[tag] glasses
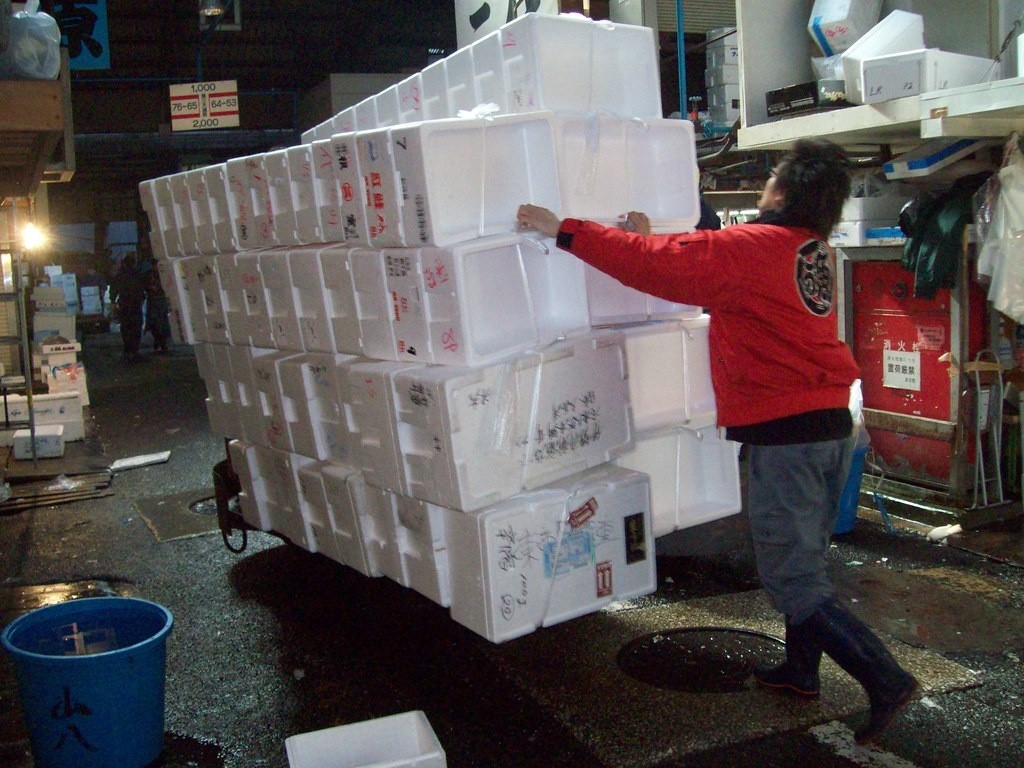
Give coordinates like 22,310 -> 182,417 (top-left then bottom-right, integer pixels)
769,168 -> 778,176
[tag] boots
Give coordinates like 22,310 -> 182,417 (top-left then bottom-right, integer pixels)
800,597 -> 920,746
754,615 -> 823,698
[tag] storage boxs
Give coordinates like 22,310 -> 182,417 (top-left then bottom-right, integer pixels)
0,266 -> 103,459
766,0 -> 994,117
136,13 -> 742,644
827,195 -> 909,249
285,710 -> 447,768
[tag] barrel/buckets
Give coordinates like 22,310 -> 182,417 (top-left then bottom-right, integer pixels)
0,596 -> 174,768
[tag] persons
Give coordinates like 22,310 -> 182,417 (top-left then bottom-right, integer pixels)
112,253 -> 174,364
517,138 -> 919,745
80,266 -> 108,331
